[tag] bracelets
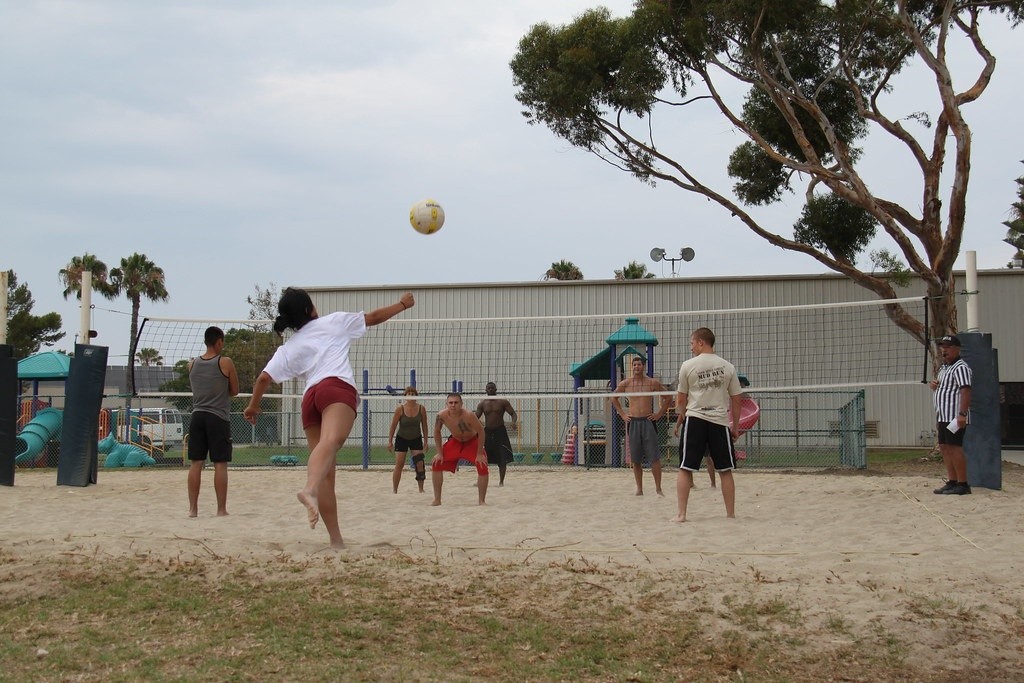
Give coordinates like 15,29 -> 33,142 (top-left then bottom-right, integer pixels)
400,301 -> 407,309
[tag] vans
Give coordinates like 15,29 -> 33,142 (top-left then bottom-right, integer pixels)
108,407 -> 183,451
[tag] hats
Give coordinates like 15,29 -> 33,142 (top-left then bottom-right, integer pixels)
935,335 -> 961,348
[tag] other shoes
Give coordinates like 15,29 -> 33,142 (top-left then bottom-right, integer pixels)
934,479 -> 958,495
942,482 -> 971,495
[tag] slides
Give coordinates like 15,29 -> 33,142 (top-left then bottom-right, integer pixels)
12,408 -> 62,469
731,395 -> 760,459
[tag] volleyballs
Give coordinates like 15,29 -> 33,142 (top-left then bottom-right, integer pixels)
409,199 -> 445,235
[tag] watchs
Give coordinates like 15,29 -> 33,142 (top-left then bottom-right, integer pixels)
959,412 -> 967,416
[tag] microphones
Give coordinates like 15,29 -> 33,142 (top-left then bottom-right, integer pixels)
943,352 -> 947,357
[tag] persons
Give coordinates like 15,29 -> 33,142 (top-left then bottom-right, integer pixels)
674,414 -> 716,488
611,357 -> 672,496
428,393 -> 489,507
388,386 -> 428,494
930,335 -> 971,495
474,382 -> 517,487
671,327 -> 742,522
245,289 -> 415,547
266,425 -> 274,446
187,327 -> 239,516
